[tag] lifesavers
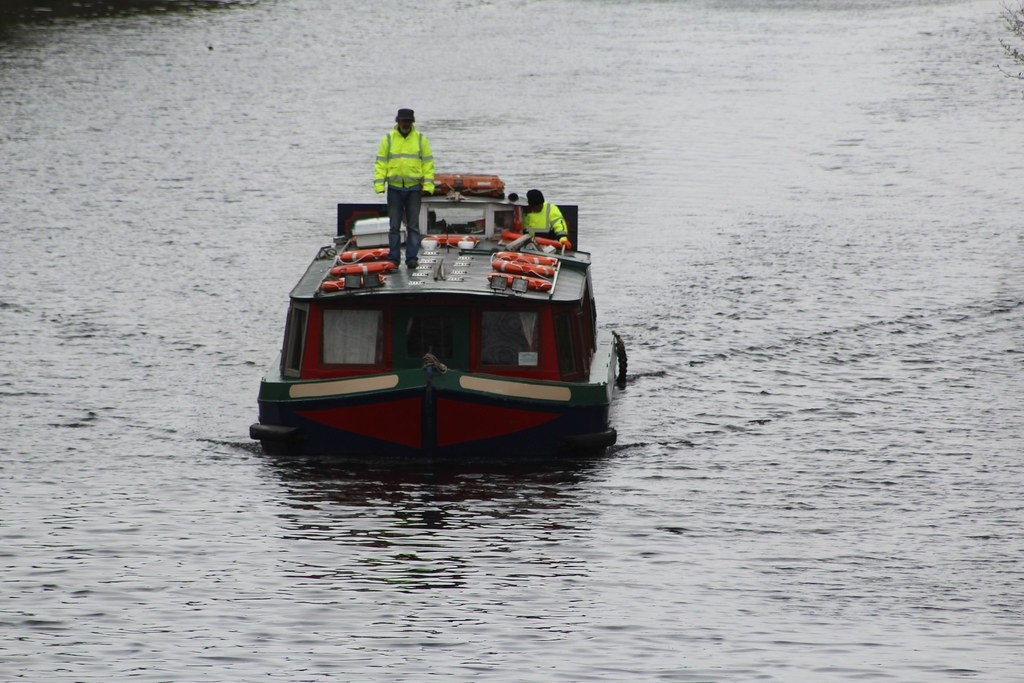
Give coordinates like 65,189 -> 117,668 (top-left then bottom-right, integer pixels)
496,250 -> 559,267
328,261 -> 397,276
493,258 -> 557,280
339,247 -> 391,261
487,272 -> 553,293
501,231 -> 563,251
425,234 -> 480,248
320,275 -> 388,291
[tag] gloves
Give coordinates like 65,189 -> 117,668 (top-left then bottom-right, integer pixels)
559,237 -> 571,249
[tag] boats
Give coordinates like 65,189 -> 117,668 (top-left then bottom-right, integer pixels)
249,174 -> 628,469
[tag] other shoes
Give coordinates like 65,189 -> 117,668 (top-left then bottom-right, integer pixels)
407,259 -> 419,269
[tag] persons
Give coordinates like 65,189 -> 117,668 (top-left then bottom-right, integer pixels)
523,189 -> 572,249
373,109 -> 435,269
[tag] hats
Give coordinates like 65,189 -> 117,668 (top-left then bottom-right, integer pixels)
526,189 -> 544,206
395,108 -> 415,122
